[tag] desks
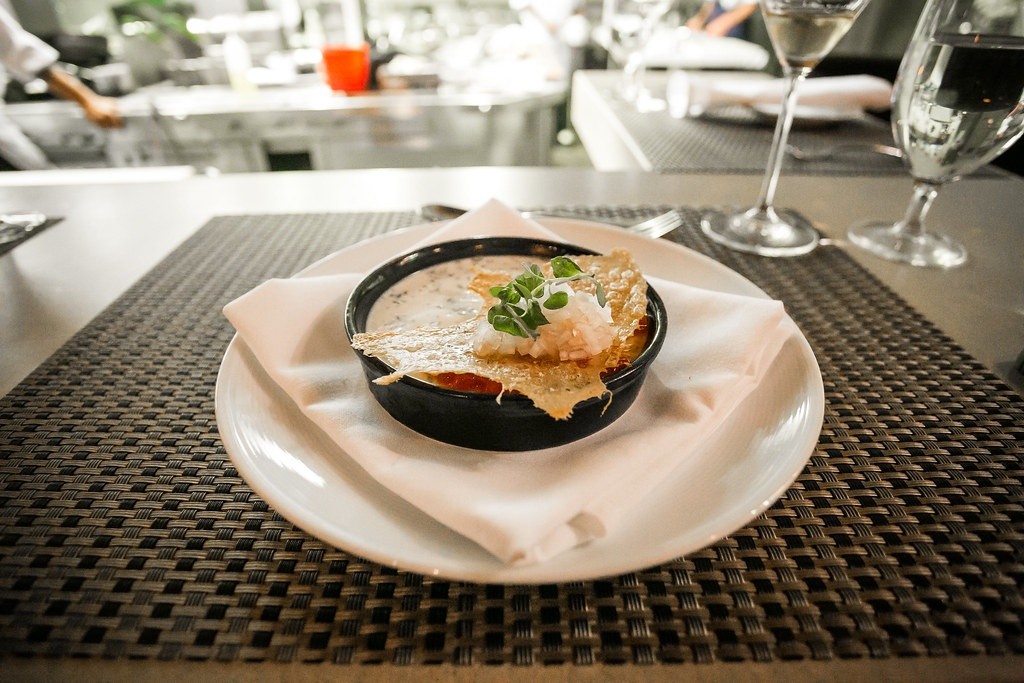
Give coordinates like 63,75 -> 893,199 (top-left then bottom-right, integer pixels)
0,72 -> 1024,683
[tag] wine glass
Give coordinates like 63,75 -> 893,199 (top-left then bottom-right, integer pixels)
847,0 -> 1024,269
702,0 -> 870,258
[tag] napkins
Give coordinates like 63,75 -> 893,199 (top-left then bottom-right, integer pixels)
667,73 -> 894,118
221,195 -> 796,568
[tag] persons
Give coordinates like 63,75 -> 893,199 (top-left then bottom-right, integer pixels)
685,0 -> 760,42
0,4 -> 128,128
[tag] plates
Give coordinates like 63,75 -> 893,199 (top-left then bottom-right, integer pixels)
215,215 -> 825,585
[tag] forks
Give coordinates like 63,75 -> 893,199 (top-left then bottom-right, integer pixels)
421,204 -> 683,238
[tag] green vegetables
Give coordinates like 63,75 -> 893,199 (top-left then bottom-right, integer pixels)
487,256 -> 607,340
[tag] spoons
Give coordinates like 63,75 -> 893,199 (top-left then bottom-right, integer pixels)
763,136 -> 904,162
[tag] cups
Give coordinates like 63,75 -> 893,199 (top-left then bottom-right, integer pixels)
320,43 -> 370,97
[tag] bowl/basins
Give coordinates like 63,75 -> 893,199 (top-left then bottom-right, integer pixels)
342,237 -> 668,453
